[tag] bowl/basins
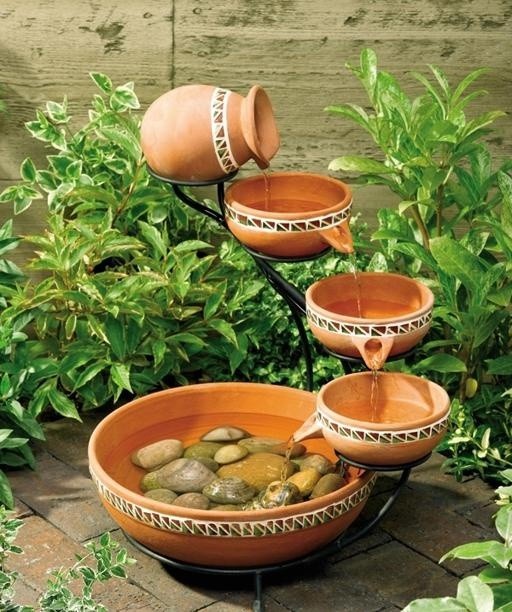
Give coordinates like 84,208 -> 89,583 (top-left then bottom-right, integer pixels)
85,381 -> 381,570
